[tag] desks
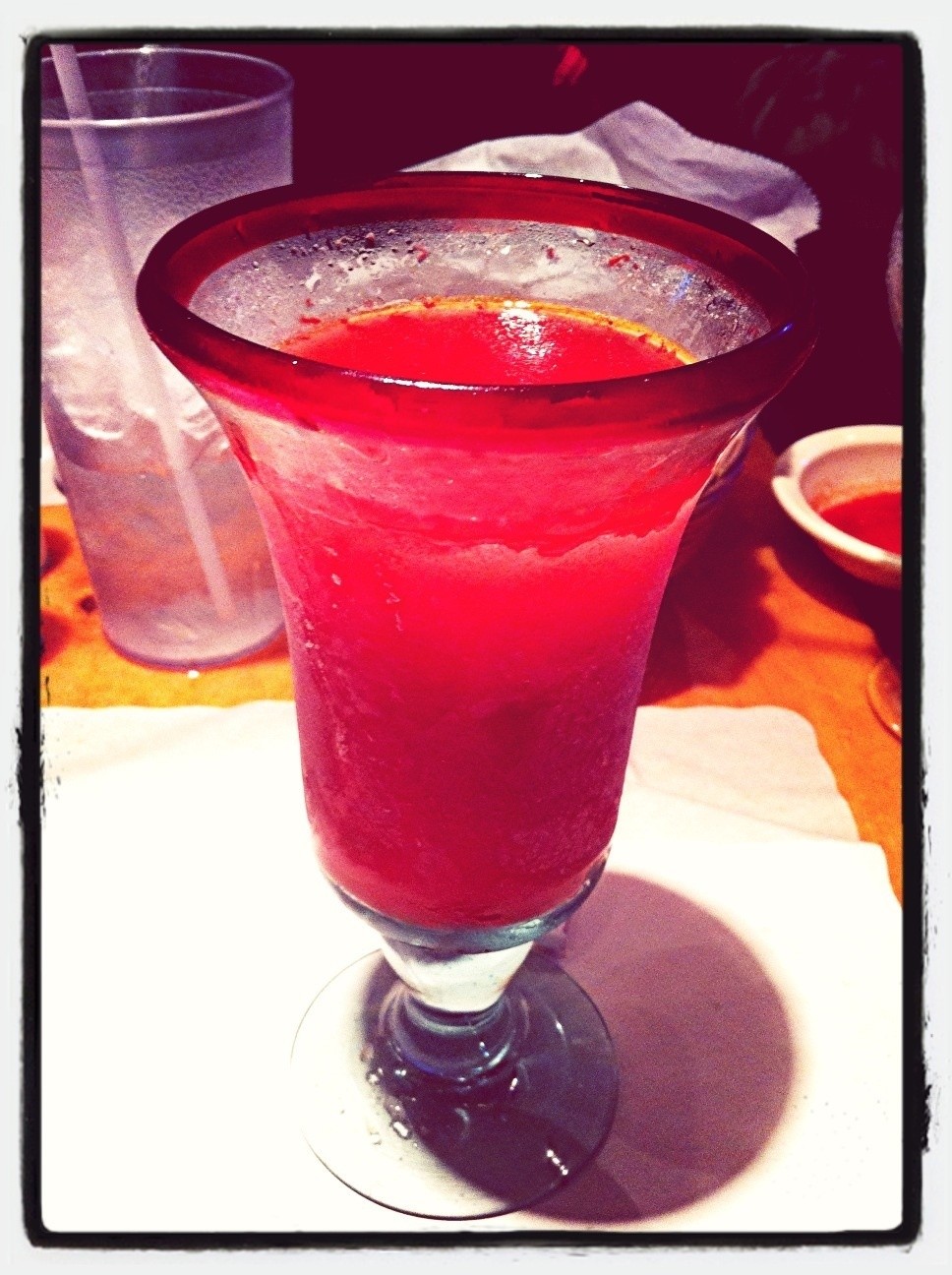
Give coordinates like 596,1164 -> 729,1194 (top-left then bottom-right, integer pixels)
40,417 -> 907,1233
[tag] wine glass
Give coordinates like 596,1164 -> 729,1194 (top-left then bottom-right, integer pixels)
135,169 -> 826,1221
859,210 -> 900,746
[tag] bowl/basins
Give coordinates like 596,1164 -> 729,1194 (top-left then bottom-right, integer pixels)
670,422 -> 750,579
770,425 -> 901,590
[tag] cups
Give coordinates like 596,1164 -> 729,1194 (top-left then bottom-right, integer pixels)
39,50 -> 294,667
